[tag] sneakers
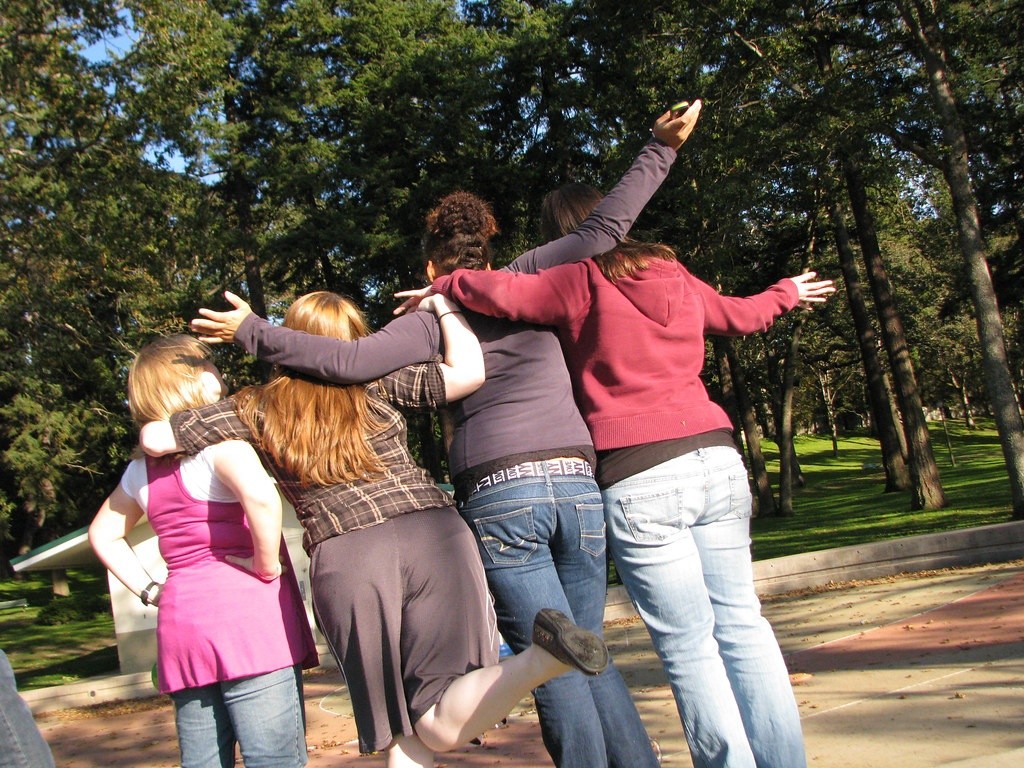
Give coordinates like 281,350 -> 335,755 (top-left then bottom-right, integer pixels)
530,608 -> 609,675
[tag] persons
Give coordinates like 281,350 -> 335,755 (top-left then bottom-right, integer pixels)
393,183 -> 835,768
139,291 -> 608,768
190,98 -> 702,766
88,335 -> 317,767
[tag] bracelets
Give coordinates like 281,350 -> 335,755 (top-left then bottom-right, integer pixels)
436,311 -> 463,322
252,561 -> 281,581
141,581 -> 158,606
